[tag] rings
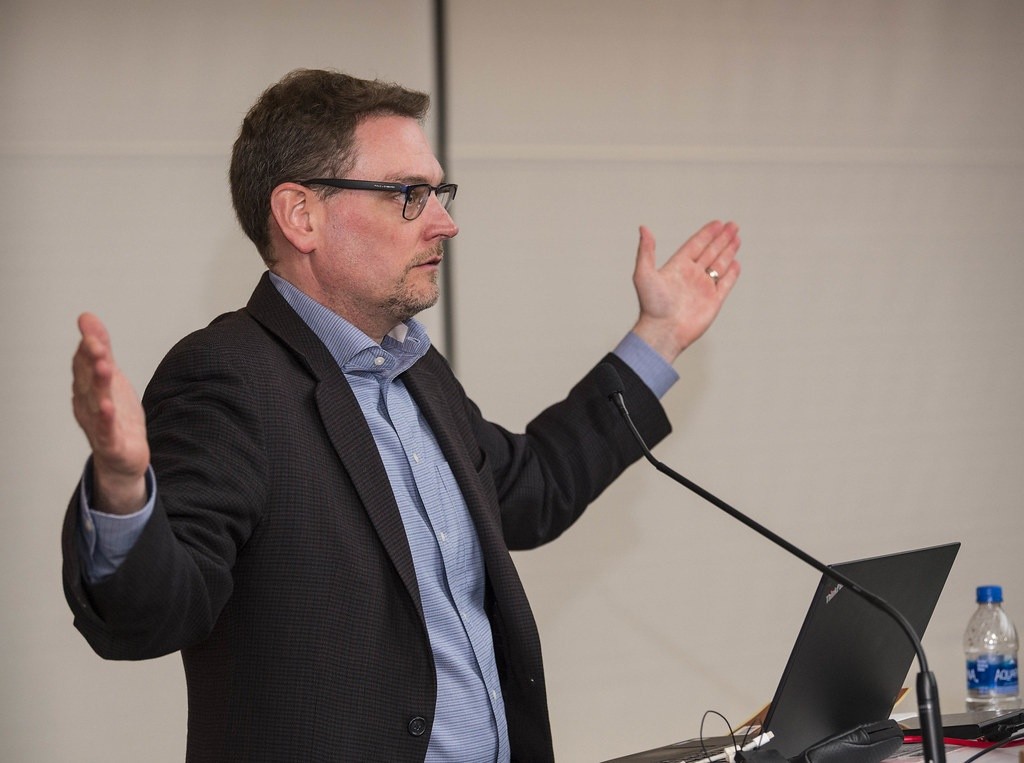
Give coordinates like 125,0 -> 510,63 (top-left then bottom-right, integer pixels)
705,267 -> 719,284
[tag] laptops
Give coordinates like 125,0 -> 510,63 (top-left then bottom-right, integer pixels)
601,543 -> 959,763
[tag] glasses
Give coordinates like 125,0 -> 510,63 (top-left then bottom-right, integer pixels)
299,178 -> 458,221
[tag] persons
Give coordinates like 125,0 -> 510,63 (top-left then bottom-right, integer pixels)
61,67 -> 740,763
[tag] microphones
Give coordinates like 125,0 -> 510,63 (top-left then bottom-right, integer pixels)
592,362 -> 947,763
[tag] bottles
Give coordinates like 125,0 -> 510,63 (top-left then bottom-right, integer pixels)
962,585 -> 1022,711
